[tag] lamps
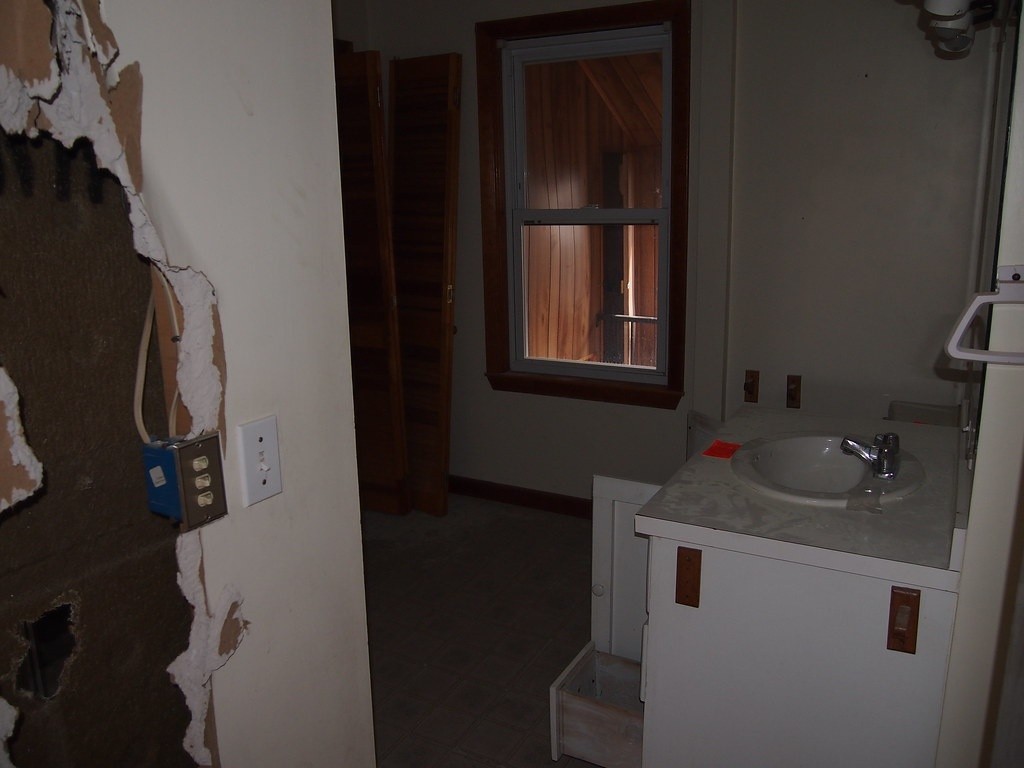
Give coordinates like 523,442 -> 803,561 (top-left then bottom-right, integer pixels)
923,0 -> 995,56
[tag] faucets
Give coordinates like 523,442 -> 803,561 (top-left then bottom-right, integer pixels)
839,432 -> 900,481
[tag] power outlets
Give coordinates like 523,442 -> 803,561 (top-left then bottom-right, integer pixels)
236,414 -> 287,508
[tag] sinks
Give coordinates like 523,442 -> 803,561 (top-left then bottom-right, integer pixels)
729,429 -> 927,507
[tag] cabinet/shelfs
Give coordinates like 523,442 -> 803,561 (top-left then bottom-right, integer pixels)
551,644 -> 643,768
591,410 -> 718,662
